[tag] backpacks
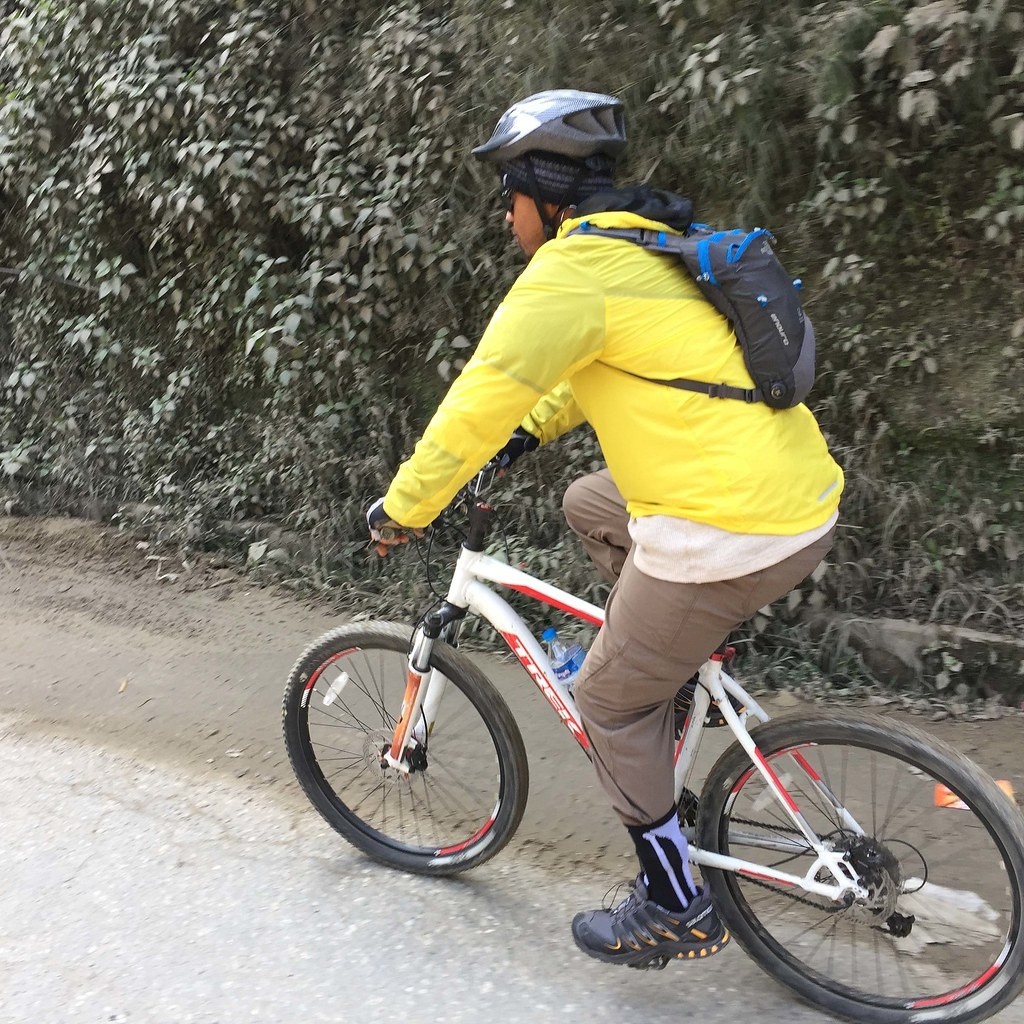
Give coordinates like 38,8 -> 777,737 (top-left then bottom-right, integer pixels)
564,220 -> 817,411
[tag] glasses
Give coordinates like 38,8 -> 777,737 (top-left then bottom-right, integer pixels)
498,187 -> 521,211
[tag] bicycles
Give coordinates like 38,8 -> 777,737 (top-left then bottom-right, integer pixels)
283,448 -> 1024,1024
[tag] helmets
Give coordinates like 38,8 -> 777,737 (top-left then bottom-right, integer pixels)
471,88 -> 629,160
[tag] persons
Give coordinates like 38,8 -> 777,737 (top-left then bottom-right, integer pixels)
366,90 -> 844,968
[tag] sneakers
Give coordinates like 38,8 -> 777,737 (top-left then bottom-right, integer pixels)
674,683 -> 748,728
572,874 -> 730,966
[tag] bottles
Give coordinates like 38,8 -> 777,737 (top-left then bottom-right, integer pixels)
541,628 -> 588,700
